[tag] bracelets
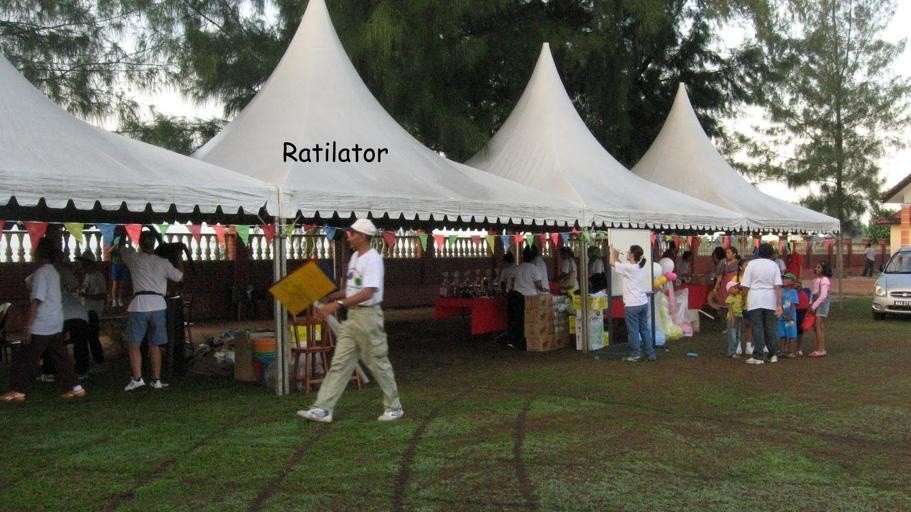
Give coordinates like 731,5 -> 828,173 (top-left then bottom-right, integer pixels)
336,300 -> 345,311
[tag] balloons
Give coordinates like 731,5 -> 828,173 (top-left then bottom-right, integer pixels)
652,258 -> 677,291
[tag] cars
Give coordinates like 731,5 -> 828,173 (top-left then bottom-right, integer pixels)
871,248 -> 911,318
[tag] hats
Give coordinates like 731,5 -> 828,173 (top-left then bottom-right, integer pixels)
782,273 -> 797,283
343,219 -> 377,237
73,250 -> 101,265
707,289 -> 723,310
726,281 -> 741,291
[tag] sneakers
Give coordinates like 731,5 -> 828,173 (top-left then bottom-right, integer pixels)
378,408 -> 405,421
1,373 -> 162,401
297,408 -> 333,423
731,344 -> 826,365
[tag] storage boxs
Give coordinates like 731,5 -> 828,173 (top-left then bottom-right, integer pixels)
688,309 -> 714,333
523,293 -> 608,353
248,331 -> 276,353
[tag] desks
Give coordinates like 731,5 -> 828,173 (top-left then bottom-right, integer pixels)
434,295 -> 509,343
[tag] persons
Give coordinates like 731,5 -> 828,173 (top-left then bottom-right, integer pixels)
296,218 -> 404,424
859,242 -> 875,277
500,243 -> 608,352
662,241 -> 677,291
609,245 -> 656,362
679,240 -> 831,365
0,230 -> 185,402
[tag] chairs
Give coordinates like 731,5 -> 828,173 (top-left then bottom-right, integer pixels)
289,315 -> 336,394
